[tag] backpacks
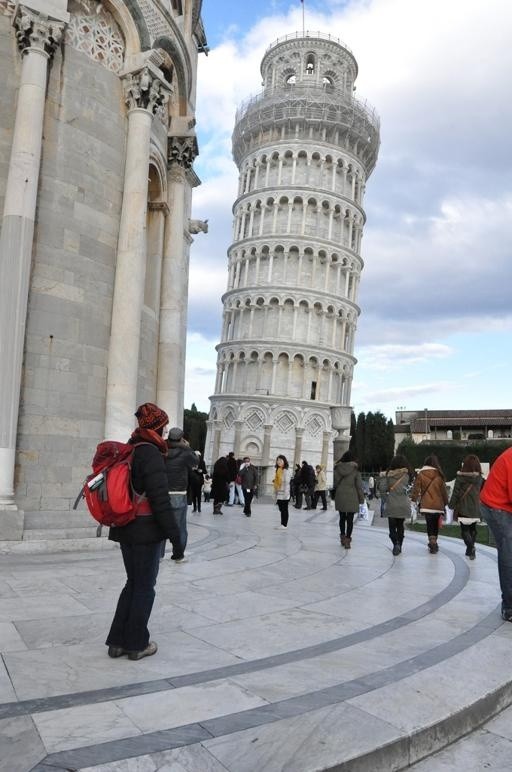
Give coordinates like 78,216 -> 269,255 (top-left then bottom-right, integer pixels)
72,441 -> 138,539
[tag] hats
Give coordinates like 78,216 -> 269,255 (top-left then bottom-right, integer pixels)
169,427 -> 185,442
134,402 -> 169,431
195,451 -> 201,456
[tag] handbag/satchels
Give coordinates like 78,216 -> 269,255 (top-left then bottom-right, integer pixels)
356,503 -> 370,522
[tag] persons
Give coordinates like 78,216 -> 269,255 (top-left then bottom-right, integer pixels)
164,427 -> 200,561
380,454 -> 411,555
447,453 -> 485,560
103,402 -> 187,661
190,448 -> 379,530
479,444 -> 512,622
332,450 -> 364,549
412,456 -> 448,554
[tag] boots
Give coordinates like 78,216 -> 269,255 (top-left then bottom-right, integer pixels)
428,535 -> 439,554
340,535 -> 352,548
462,531 -> 476,560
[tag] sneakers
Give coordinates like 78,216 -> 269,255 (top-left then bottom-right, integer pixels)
275,524 -> 289,530
128,641 -> 158,661
392,540 -> 402,555
108,645 -> 125,658
